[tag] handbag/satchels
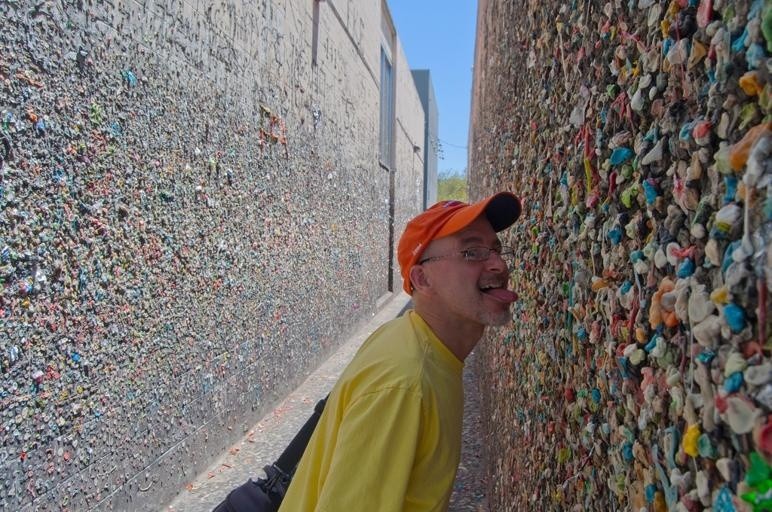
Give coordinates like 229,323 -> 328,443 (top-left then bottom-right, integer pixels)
212,465 -> 291,512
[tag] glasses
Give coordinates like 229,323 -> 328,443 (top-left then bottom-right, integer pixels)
418,246 -> 515,265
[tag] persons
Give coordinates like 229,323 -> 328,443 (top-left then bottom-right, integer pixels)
277,191 -> 521,512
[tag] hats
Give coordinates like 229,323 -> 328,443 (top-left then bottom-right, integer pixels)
398,191 -> 521,296
